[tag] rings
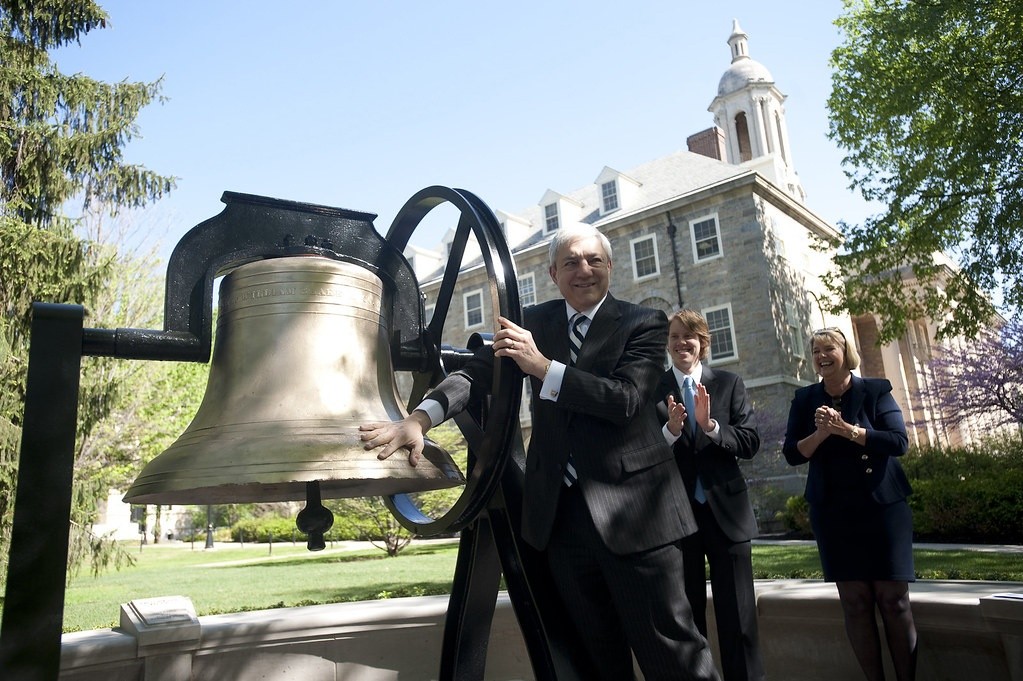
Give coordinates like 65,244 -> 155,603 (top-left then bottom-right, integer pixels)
511,340 -> 515,348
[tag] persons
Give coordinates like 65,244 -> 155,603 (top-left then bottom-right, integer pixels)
359,222 -> 722,681
655,308 -> 765,681
781,326 -> 917,681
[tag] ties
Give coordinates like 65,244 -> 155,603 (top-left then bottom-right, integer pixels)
563,312 -> 586,488
683,376 -> 708,504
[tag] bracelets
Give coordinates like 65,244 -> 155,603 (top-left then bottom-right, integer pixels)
542,361 -> 552,382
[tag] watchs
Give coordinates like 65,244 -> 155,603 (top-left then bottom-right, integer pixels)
849,423 -> 860,441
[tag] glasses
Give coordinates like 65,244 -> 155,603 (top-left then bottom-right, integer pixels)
817,327 -> 842,332
